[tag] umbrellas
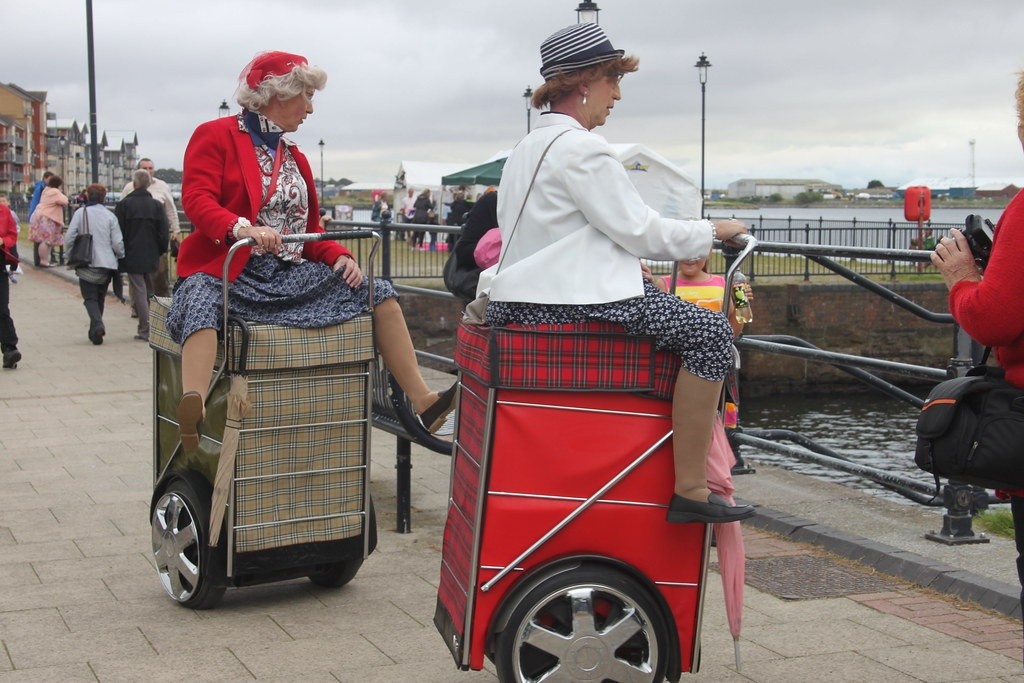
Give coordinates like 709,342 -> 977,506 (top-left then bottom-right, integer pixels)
706,343 -> 745,672
205,315 -> 252,573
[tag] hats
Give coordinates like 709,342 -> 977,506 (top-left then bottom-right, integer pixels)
238,50 -> 308,89
540,22 -> 625,81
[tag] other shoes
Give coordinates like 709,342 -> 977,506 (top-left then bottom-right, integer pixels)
88,329 -> 105,344
131,308 -> 139,317
3,348 -> 21,368
421,379 -> 461,433
41,262 -> 57,268
177,390 -> 206,450
135,333 -> 146,340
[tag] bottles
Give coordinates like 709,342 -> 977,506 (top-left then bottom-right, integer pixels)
732,267 -> 753,324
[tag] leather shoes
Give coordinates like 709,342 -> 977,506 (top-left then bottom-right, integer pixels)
664,489 -> 758,523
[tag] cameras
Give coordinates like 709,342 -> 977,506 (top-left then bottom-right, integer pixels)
935,214 -> 996,276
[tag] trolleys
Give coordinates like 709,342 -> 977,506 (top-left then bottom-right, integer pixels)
147,227 -> 384,613
429,227 -> 762,683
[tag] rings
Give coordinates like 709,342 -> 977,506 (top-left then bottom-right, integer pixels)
730,218 -> 736,221
260,232 -> 266,237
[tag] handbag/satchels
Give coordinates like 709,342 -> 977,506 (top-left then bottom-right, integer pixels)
913,364 -> 1024,489
68,207 -> 93,269
443,236 -> 483,300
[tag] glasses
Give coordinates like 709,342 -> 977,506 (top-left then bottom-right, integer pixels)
604,71 -> 623,83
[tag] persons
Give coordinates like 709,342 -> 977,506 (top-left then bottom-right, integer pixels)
27,172 -> 69,268
914,74 -> 1024,625
119,159 -> 181,317
0,194 -> 22,369
63,183 -> 125,345
372,186 -> 500,251
482,24 -> 756,524
166,55 -> 458,448
113,169 -> 170,341
639,243 -> 754,469
62,188 -> 108,220
909,225 -> 937,268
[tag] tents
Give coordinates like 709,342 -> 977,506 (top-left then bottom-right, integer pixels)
375,151 -> 512,243
608,142 -> 704,270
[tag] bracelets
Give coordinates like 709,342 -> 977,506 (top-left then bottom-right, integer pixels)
706,219 -> 717,243
228,217 -> 252,242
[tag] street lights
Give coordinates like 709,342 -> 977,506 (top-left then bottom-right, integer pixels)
318,139 -> 325,207
522,84 -> 533,134
694,51 -> 712,219
